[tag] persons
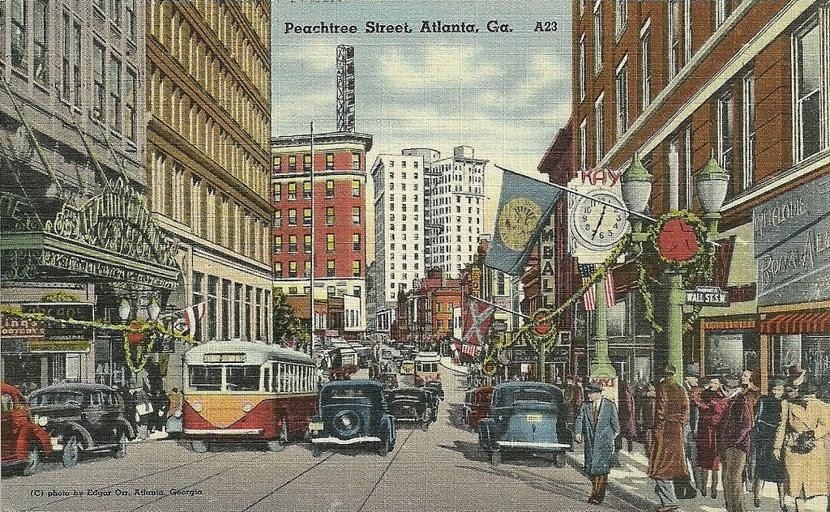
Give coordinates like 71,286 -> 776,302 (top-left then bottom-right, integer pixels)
115,384 -> 182,440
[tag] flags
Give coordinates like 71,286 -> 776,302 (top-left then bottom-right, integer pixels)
461,297 -> 496,347
165,305 -> 205,340
482,170 -> 570,279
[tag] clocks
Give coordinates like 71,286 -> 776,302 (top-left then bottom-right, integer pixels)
567,168 -> 633,265
